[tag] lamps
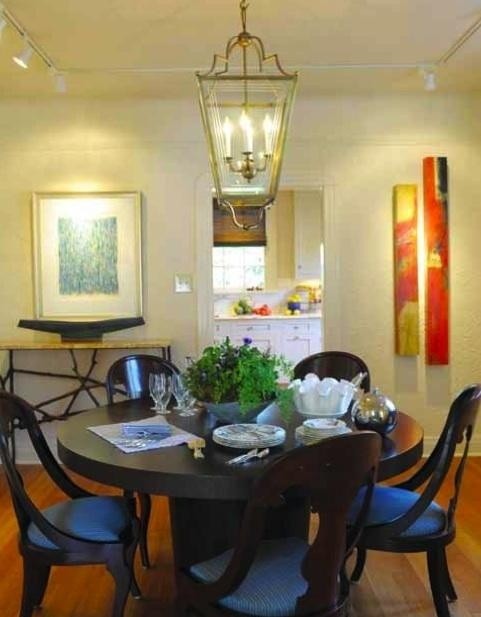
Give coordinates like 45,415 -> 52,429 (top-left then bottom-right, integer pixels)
0,0 -> 69,94
195,0 -> 298,232
422,66 -> 438,91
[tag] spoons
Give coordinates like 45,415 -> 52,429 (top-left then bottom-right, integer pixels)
226,448 -> 258,465
238,448 -> 270,464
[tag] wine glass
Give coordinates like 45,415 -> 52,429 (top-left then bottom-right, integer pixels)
147,372 -> 198,414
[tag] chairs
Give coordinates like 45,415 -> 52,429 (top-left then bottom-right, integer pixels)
346,383 -> 481,617
107,354 -> 180,569
0,390 -> 142,617
174,430 -> 382,617
293,351 -> 370,393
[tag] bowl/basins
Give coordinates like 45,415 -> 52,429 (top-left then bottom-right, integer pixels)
303,419 -> 345,432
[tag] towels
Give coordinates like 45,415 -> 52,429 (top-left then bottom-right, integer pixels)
86,415 -> 200,453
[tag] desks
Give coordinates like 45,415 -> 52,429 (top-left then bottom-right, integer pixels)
57,382 -> 423,588
0,340 -> 171,464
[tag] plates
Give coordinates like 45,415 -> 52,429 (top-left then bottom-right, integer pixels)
295,425 -> 353,445
213,424 -> 287,449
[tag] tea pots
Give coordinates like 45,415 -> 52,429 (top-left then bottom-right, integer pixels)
380,434 -> 396,459
350,386 -> 398,437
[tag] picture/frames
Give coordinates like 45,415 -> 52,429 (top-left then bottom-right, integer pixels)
32,191 -> 142,322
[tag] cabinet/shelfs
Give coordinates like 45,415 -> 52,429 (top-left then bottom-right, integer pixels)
214,318 -> 321,365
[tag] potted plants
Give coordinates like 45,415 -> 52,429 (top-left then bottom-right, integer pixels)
180,335 -> 294,424
